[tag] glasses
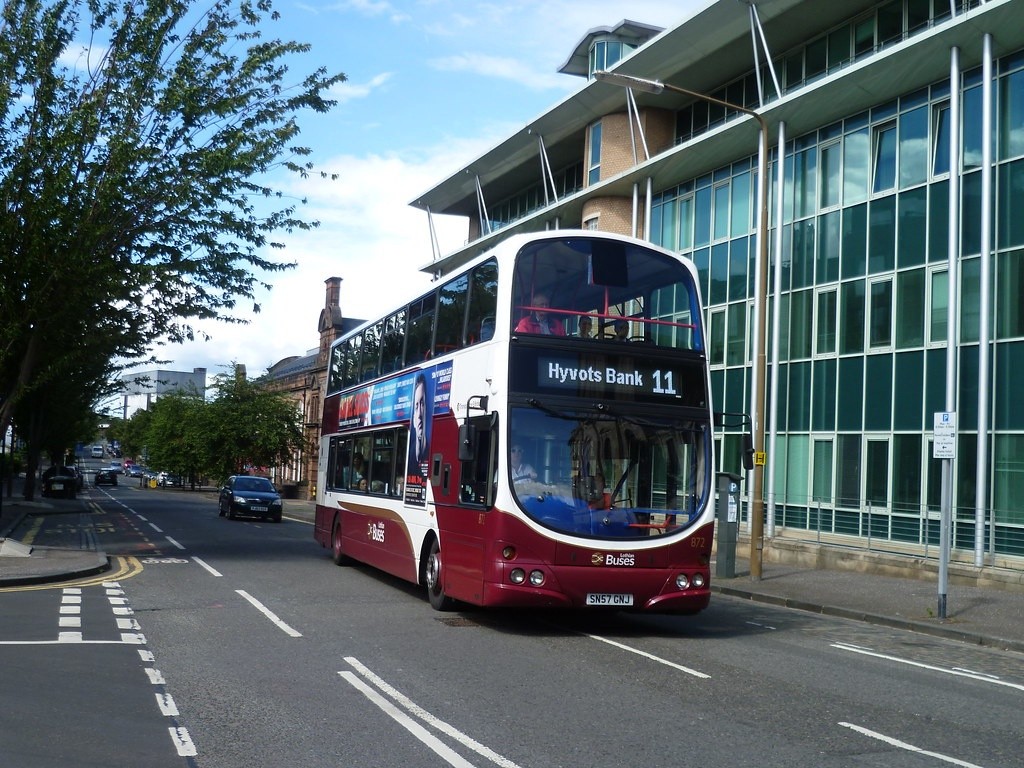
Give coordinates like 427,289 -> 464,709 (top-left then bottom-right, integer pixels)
534,303 -> 549,308
511,449 -> 523,453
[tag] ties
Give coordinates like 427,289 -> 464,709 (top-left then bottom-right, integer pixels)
418,438 -> 422,456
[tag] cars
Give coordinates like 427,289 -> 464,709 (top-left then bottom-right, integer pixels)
110,461 -> 123,473
95,468 -> 117,486
220,476 -> 283,522
41,464 -> 83,498
107,446 -> 181,488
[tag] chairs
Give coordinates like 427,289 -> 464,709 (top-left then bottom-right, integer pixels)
480,316 -> 496,341
363,354 -> 424,381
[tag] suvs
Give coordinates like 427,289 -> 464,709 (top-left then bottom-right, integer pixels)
91,445 -> 104,458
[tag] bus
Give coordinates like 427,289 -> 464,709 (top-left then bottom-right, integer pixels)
314,230 -> 713,618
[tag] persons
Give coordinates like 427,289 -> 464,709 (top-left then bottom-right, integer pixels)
572,316 -> 593,338
514,293 -> 566,337
347,452 -> 404,496
494,437 -> 538,488
610,320 -> 631,342
408,374 -> 430,482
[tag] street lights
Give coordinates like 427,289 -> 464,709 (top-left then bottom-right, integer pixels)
592,70 -> 767,585
